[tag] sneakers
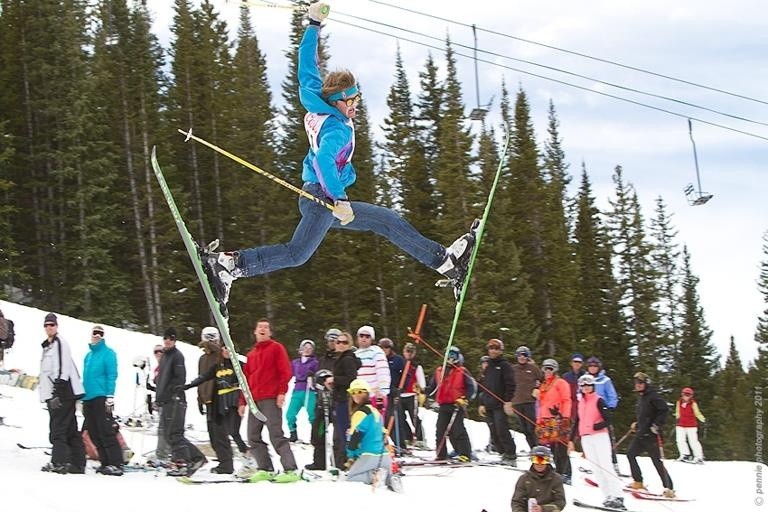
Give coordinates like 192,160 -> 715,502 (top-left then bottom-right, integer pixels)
395,432 -> 426,457
436,232 -> 475,279
623,481 -> 645,492
43,448 -> 297,482
690,457 -> 704,465
498,457 -> 518,468
390,475 -> 404,493
289,430 -> 297,442
449,454 -> 471,463
372,468 -> 388,487
561,473 -> 572,486
676,454 -> 692,462
661,489 -> 676,498
484,444 -> 497,451
638,484 -> 649,494
209,250 -> 246,304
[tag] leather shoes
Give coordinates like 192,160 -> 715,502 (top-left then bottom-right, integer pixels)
305,463 -> 324,471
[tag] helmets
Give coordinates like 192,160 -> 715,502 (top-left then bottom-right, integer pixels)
515,346 -> 532,359
634,372 -> 651,385
444,346 -> 463,364
480,355 -> 490,364
299,326 -> 416,357
529,446 -> 554,458
682,388 -> 694,394
541,353 -> 602,387
486,339 -> 505,351
202,327 -> 219,342
346,380 -> 370,392
313,369 -> 334,391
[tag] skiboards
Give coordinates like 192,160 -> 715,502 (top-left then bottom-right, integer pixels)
18,443 -> 53,456
393,446 -> 530,471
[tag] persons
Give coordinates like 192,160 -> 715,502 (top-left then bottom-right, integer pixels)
0,308 -> 18,427
671,387 -> 707,465
625,372 -> 676,499
39,312 -> 87,474
82,325 -> 125,476
76,395 -> 135,466
202,0 -> 476,305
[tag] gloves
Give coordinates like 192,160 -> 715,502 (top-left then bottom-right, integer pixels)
48,397 -> 64,410
454,396 -> 469,407
503,402 -> 513,415
104,399 -> 115,413
534,425 -> 543,438
308,1 -> 330,27
532,388 -> 541,399
332,200 -> 352,222
478,405 -> 488,418
198,403 -> 206,414
559,420 -> 572,434
173,384 -> 191,392
412,383 -> 423,395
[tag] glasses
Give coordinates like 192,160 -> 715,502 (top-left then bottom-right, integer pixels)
349,390 -> 366,395
92,330 -> 105,338
43,323 -> 56,327
164,335 -> 176,340
681,392 -> 691,397
154,350 -> 164,354
338,94 -> 361,108
222,346 -> 228,352
530,455 -> 551,465
634,379 -> 651,385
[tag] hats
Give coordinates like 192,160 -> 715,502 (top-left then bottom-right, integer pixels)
45,313 -> 57,324
165,328 -> 177,337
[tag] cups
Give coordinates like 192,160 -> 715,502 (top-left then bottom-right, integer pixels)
527,497 -> 537,512
